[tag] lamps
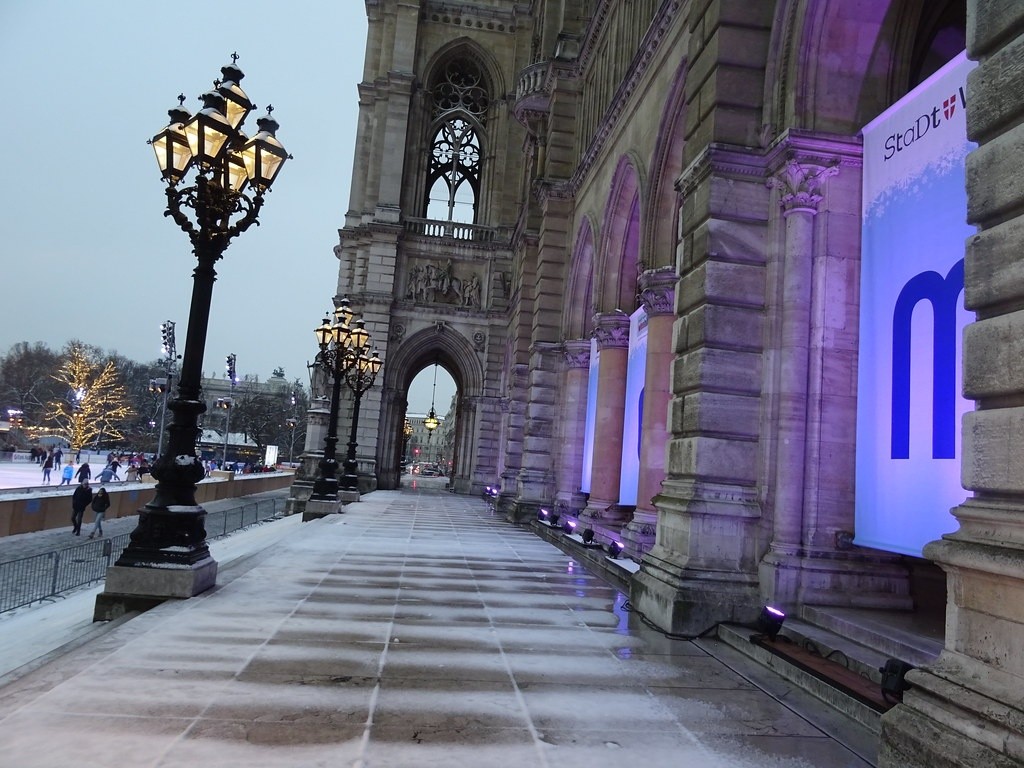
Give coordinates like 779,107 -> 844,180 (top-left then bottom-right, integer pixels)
757,605 -> 786,636
485,486 -> 498,494
562,522 -> 576,534
422,408 -> 440,438
549,513 -> 560,525
537,508 -> 549,520
608,541 -> 625,559
878,659 -> 916,701
582,529 -> 594,544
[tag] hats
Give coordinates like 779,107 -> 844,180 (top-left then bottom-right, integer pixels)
100,488 -> 105,491
132,465 -> 135,468
82,479 -> 88,485
69,461 -> 74,465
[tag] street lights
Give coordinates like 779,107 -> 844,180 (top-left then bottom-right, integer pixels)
401,418 -> 412,466
308,297 -> 371,504
223,462 -> 225,465
342,340 -> 384,494
149,318 -> 177,459
98,46 -> 288,599
285,388 -> 298,466
221,353 -> 237,471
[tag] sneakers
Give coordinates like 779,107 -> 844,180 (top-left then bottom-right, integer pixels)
98,531 -> 102,537
73,528 -> 80,536
88,533 -> 94,538
42,480 -> 50,485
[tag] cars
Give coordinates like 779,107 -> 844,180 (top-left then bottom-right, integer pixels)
423,469 -> 434,476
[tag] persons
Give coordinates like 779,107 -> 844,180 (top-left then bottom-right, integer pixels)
31,445 -> 62,470
42,456 -> 54,486
75,464 -> 91,484
95,451 -> 154,484
58,460 -> 73,485
203,456 -> 276,476
70,478 -> 93,534
88,487 -> 110,540
